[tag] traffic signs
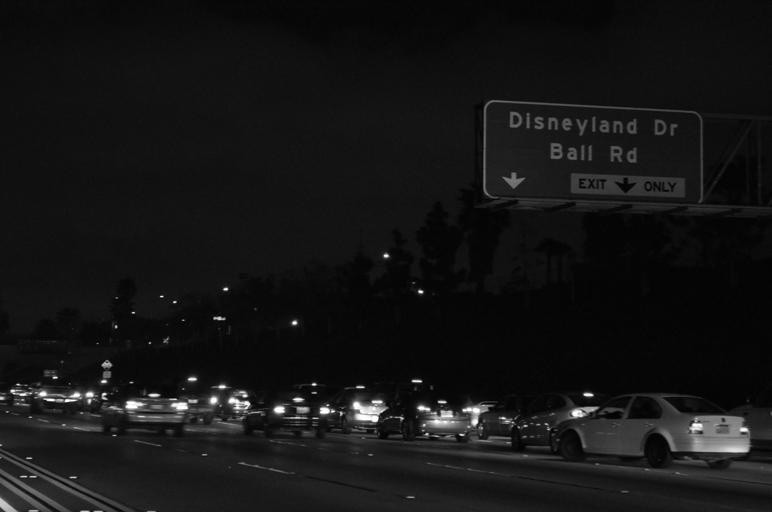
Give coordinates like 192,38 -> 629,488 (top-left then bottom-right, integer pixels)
482,100 -> 703,207
